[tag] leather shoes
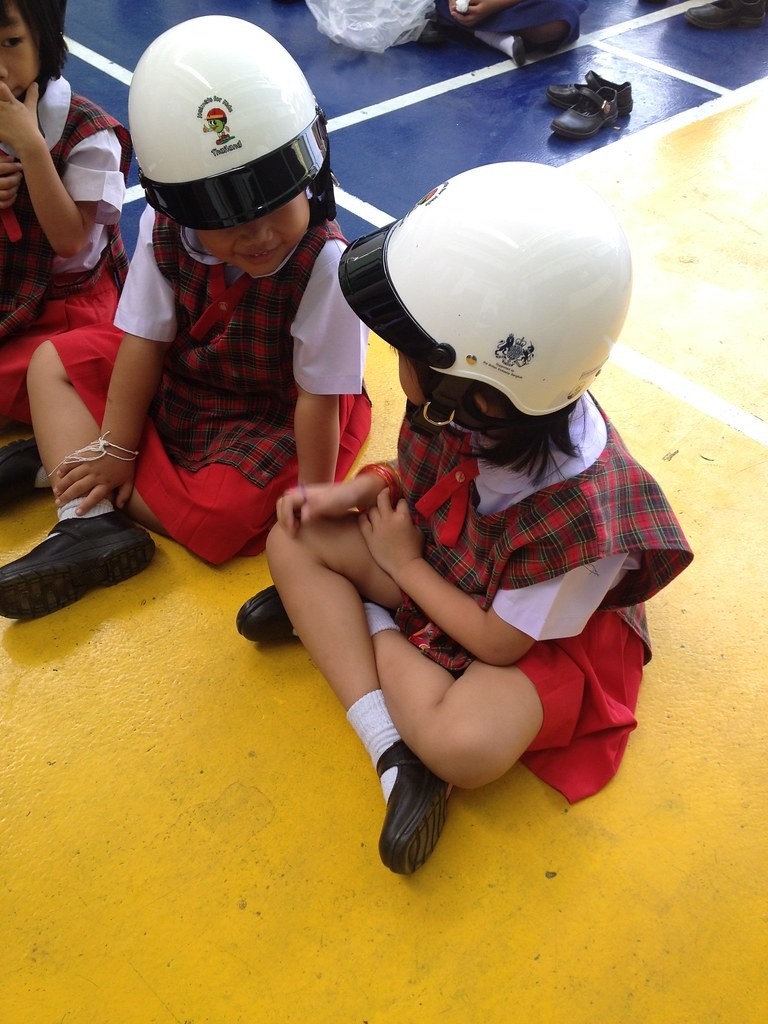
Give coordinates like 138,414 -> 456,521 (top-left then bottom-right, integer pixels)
0,435 -> 52,506
237,584 -> 294,651
376,739 -> 459,877
0,505 -> 156,622
546,71 -> 632,139
684,0 -> 765,29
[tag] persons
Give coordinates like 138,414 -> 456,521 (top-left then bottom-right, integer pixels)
0,0 -> 134,434
305,0 -> 590,66
236,161 -> 694,875
0,14 -> 372,620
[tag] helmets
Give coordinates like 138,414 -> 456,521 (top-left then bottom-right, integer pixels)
128,14 -> 330,229
338,160 -> 633,415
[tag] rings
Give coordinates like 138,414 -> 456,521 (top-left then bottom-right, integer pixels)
300,483 -> 307,505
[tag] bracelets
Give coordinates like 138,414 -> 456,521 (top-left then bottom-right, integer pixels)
354,460 -> 403,514
47,430 -> 139,478
